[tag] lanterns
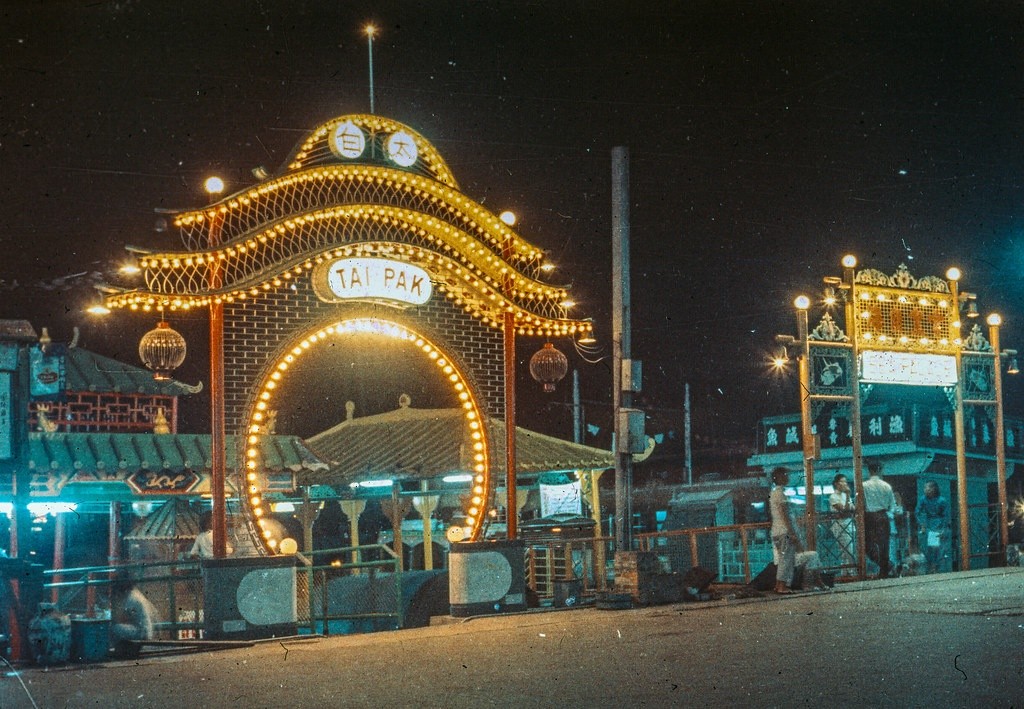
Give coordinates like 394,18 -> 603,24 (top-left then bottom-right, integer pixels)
530,342 -> 568,392
138,321 -> 186,380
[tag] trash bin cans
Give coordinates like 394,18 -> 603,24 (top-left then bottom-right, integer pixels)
551,578 -> 581,608
70,617 -> 112,661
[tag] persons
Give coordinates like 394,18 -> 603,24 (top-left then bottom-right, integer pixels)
862,463 -> 900,576
190,511 -> 234,561
888,490 -> 904,577
827,474 -> 861,577
769,546 -> 831,591
111,572 -> 153,661
913,479 -> 950,574
768,467 -> 798,594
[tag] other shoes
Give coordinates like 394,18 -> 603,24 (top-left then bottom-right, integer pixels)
773,586 -> 795,595
816,585 -> 832,591
803,585 -> 821,593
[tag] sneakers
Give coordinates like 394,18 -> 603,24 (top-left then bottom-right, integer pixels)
878,561 -> 895,578
